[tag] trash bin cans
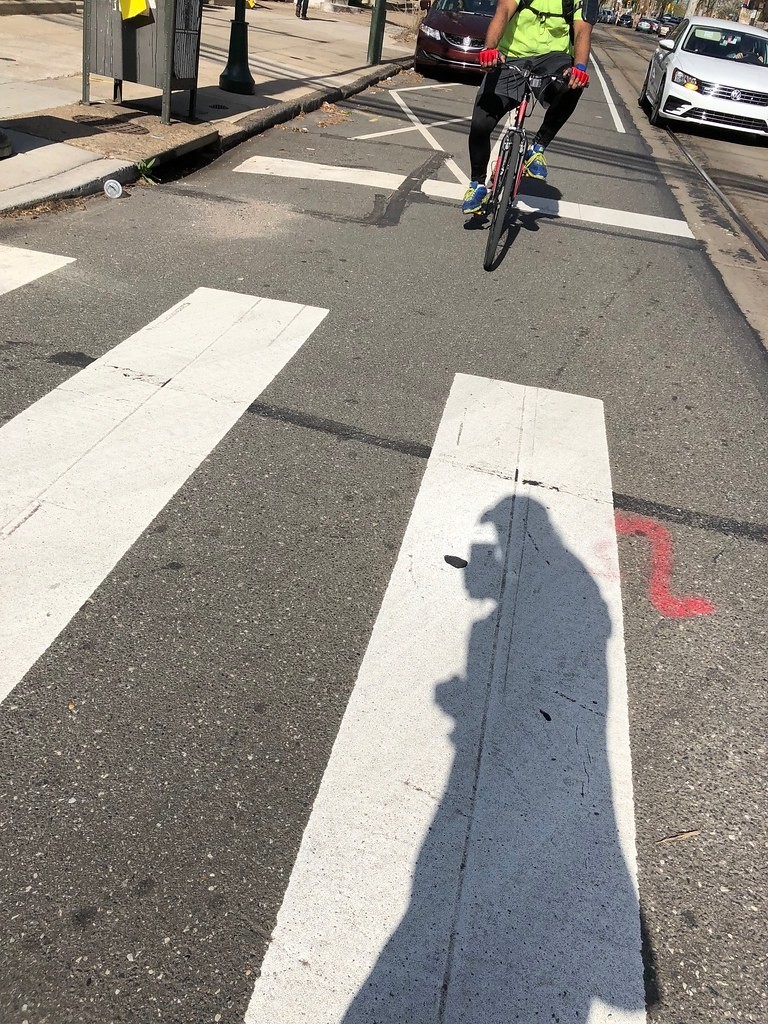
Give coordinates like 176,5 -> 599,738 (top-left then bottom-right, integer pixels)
77,0 -> 207,125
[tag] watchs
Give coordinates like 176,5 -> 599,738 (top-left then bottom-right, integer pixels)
575,63 -> 587,72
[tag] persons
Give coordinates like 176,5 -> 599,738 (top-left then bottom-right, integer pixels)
295,0 -> 309,20
460,0 -> 598,215
725,36 -> 764,63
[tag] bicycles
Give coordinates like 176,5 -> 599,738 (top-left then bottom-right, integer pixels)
478,59 -> 591,269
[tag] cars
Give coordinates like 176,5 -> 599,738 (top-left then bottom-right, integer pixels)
641,17 -> 768,142
595,9 -> 682,39
412,0 -> 505,76
